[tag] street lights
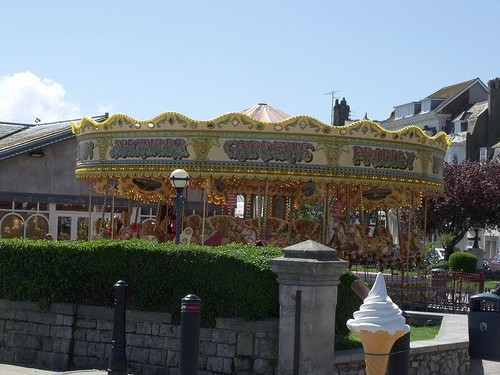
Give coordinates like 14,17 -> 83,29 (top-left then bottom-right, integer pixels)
472,225 -> 480,248
170,169 -> 190,245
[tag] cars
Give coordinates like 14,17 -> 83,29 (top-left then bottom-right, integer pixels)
482,254 -> 500,270
435,248 -> 446,260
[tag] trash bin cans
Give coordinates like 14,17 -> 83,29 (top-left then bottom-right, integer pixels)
467,292 -> 500,363
